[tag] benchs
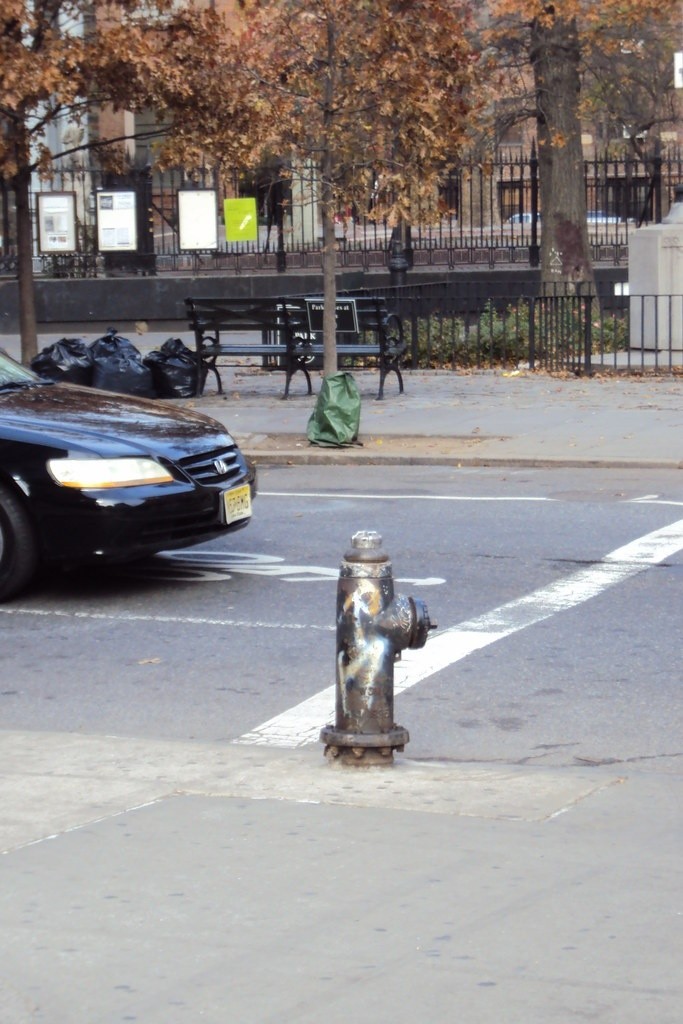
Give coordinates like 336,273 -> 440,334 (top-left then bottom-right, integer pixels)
182,295 -> 409,401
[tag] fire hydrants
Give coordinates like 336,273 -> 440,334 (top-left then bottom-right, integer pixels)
317,530 -> 439,765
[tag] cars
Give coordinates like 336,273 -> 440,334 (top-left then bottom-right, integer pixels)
1,342 -> 257,606
